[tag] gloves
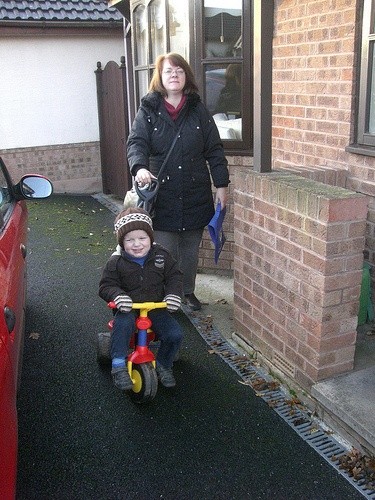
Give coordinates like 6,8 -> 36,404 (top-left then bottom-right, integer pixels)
113,295 -> 133,314
163,295 -> 182,313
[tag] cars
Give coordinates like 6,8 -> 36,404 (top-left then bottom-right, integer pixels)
0,158 -> 56,500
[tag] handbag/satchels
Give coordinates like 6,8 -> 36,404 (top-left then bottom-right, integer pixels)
208,203 -> 226,264
137,190 -> 156,218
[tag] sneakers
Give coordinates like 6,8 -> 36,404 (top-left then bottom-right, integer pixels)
184,294 -> 202,312
111,368 -> 134,390
157,363 -> 176,387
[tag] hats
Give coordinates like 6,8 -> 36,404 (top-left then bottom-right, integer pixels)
114,207 -> 154,249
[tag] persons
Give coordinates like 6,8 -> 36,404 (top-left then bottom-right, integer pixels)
127,52 -> 230,312
100,206 -> 181,390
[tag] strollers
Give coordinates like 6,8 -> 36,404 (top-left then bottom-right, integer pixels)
96,178 -> 179,406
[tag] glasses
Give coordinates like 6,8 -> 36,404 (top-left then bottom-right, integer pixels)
160,70 -> 186,75
